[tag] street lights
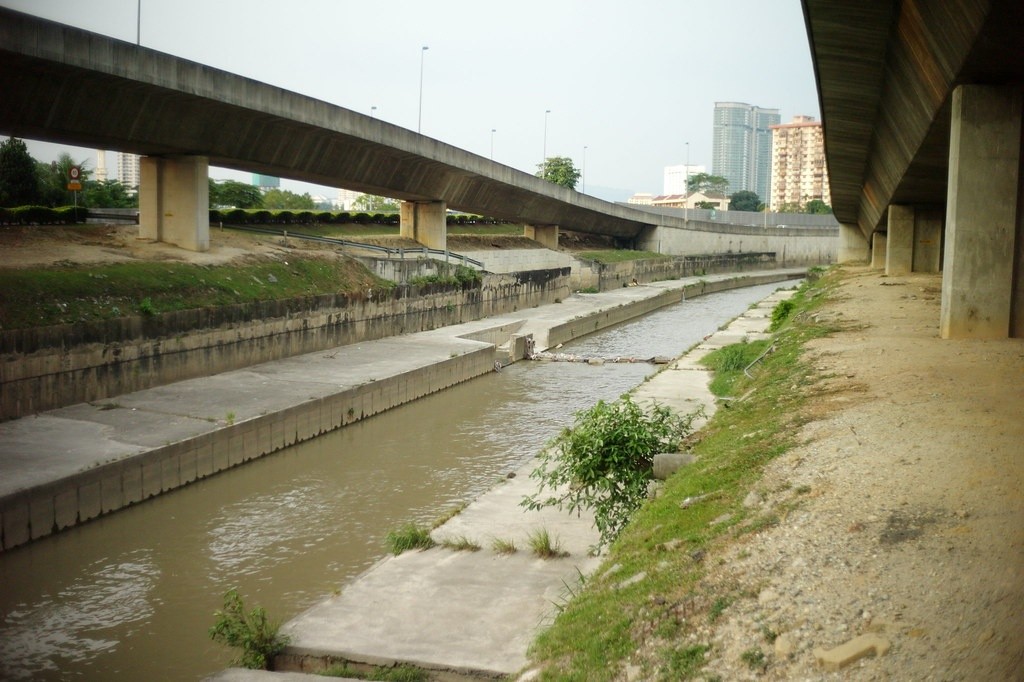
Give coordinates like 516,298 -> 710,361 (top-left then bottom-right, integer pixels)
685,142 -> 688,222
542,110 -> 551,179
370,106 -> 376,117
418,46 -> 429,135
583,145 -> 588,194
491,129 -> 496,160
